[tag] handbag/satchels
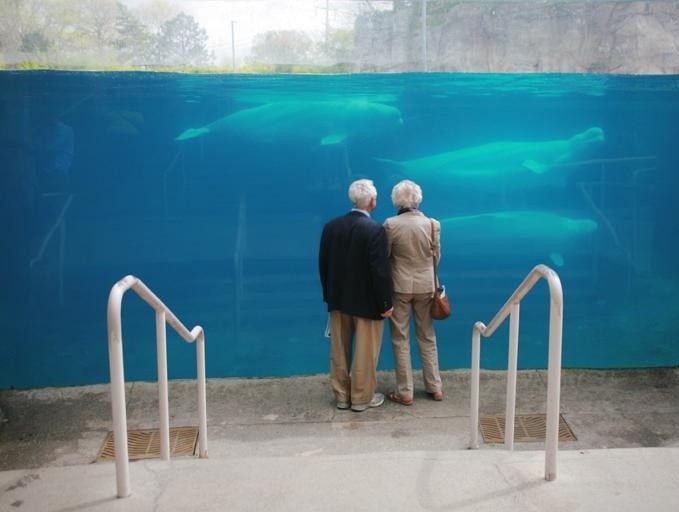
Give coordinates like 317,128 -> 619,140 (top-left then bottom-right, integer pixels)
431,287 -> 450,319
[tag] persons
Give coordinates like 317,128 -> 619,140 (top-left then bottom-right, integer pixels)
382,179 -> 446,406
318,179 -> 394,412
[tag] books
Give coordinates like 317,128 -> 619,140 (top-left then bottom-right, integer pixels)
325,312 -> 331,338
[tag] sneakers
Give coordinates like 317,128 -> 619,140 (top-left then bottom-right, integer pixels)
351,393 -> 385,410
386,389 -> 414,405
336,400 -> 351,409
433,390 -> 443,401
